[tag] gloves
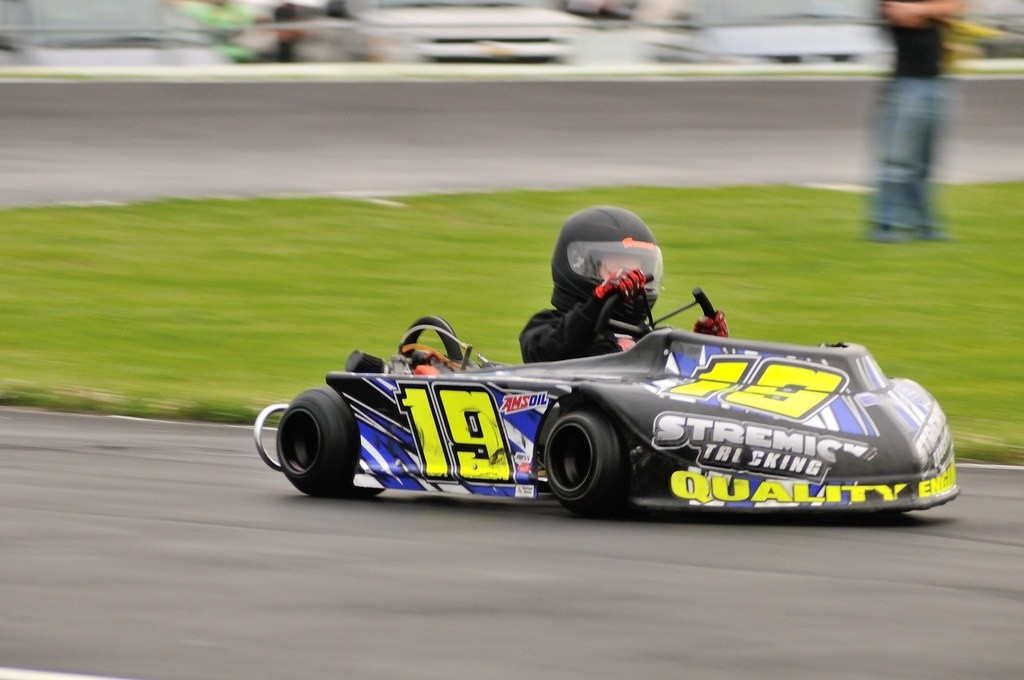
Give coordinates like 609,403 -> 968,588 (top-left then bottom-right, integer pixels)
693,310 -> 728,337
594,267 -> 647,300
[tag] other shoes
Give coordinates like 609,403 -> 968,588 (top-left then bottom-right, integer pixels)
918,223 -> 954,244
866,224 -> 906,246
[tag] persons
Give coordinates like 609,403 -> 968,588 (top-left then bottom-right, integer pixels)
519,206 -> 728,364
164,0 -> 358,64
862,0 -> 969,243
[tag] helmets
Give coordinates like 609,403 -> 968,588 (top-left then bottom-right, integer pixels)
551,206 -> 658,334
